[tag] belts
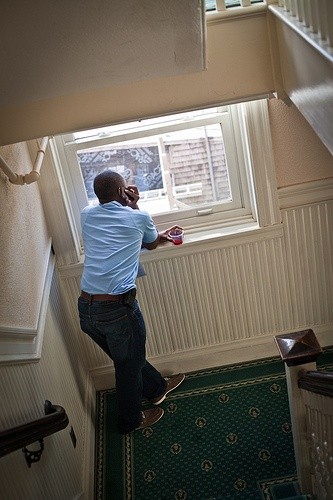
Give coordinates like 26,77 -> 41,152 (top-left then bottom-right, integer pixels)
80,290 -> 119,302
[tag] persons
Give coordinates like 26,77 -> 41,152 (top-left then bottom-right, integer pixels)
77,170 -> 186,436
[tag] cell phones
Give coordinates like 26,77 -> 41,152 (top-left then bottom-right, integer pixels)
118,187 -> 134,201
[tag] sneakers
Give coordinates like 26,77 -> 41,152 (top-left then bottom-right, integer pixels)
129,408 -> 164,431
153,373 -> 185,406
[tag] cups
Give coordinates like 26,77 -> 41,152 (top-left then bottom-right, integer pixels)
168,229 -> 184,246
